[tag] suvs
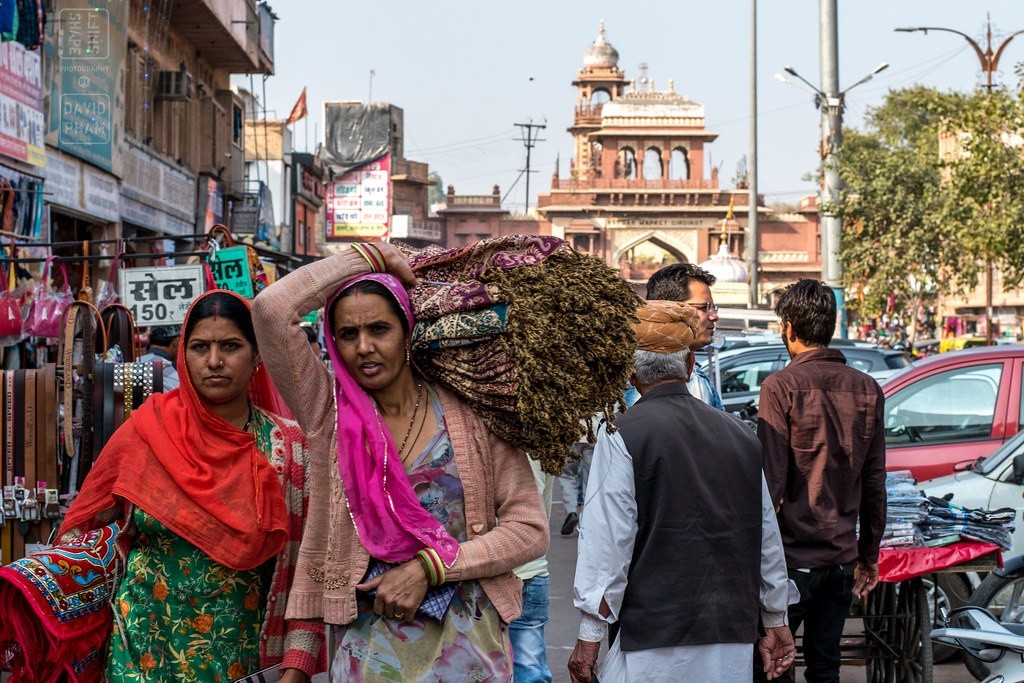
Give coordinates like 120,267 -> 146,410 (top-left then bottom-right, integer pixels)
696,336 -> 1002,442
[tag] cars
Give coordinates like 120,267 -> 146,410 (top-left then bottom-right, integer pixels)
844,425 -> 1024,664
876,347 -> 1024,482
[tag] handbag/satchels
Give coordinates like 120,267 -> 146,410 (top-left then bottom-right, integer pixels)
0,263 -> 22,336
23,255 -> 75,338
97,253 -> 151,343
79,241 -> 94,318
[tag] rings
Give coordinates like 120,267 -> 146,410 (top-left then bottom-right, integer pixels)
394,613 -> 403,618
785,656 -> 787,658
779,659 -> 782,661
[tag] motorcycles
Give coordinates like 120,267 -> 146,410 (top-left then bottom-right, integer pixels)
939,338 -> 997,356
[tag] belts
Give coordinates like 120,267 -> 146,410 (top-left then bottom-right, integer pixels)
1,300 -> 163,565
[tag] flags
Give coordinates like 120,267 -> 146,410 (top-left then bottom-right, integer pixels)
285,90 -> 310,125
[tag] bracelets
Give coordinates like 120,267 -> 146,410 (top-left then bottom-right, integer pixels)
417,548 -> 446,586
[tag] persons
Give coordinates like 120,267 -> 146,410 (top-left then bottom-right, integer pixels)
567,300 -> 800,683
751,279 -> 887,683
0,289 -> 327,683
619,262 -> 724,411
251,241 -> 594,683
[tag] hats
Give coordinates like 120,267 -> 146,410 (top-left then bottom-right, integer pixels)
631,300 -> 700,353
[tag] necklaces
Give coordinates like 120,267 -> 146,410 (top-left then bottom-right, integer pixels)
397,380 -> 429,464
242,404 -> 252,431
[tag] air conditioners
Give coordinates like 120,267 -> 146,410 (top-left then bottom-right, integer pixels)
154,69 -> 192,100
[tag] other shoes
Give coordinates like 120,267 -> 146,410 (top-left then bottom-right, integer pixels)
561,512 -> 579,535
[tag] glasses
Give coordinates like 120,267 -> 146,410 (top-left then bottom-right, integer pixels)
687,302 -> 719,312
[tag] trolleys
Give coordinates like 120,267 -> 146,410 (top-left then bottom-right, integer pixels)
753,546 -> 998,683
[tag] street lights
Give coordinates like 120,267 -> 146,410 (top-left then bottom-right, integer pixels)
786,63 -> 889,339
895,27 -> 1024,355
